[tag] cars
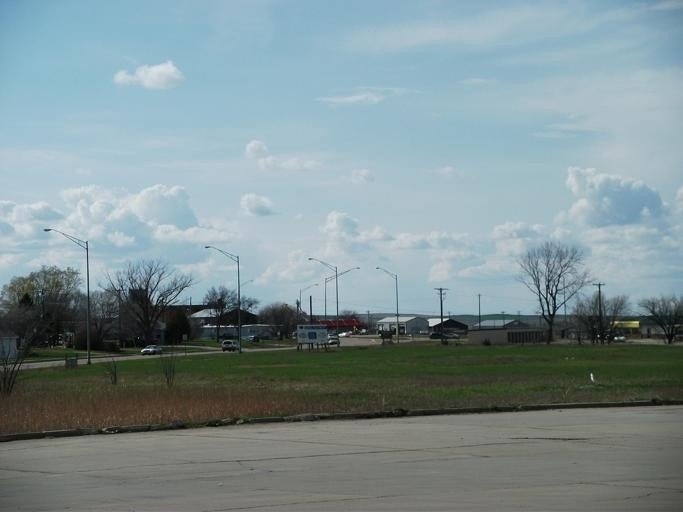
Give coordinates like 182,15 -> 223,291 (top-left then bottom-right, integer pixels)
140,344 -> 162,355
428,332 -> 459,339
328,333 -> 340,345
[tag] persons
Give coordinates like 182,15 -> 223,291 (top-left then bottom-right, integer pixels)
565,327 -> 621,345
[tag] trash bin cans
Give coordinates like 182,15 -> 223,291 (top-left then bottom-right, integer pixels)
66,357 -> 77,366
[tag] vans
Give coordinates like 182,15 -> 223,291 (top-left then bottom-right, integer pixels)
220,339 -> 240,352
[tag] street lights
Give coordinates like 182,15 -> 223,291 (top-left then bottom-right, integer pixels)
324,267 -> 360,325
203,245 -> 241,351
375,265 -> 399,343
42,227 -> 89,364
299,283 -> 318,324
307,257 -> 339,348
